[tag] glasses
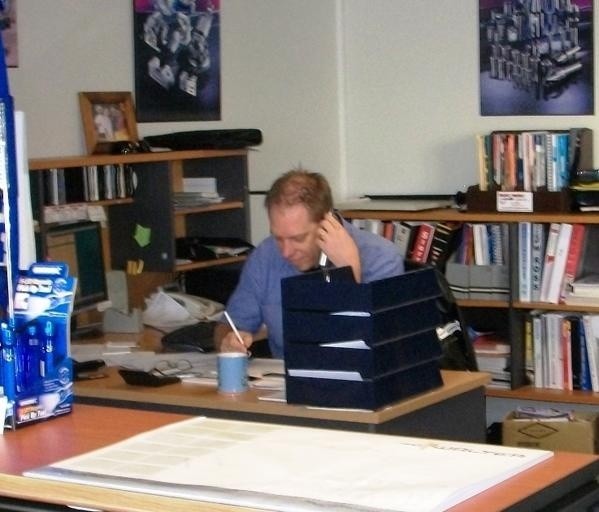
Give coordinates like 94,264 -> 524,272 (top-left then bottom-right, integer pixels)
147,359 -> 193,377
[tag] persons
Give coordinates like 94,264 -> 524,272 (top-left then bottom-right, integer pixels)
93,103 -> 128,140
212,167 -> 406,357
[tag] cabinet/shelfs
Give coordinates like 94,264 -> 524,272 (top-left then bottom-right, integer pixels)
333,199 -> 599,401
27,149 -> 253,352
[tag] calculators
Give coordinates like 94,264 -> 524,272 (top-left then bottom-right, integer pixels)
119,369 -> 181,386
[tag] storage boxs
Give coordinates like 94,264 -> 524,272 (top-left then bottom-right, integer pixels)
501,406 -> 599,454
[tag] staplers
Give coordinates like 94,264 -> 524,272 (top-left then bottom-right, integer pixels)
74,358 -> 106,380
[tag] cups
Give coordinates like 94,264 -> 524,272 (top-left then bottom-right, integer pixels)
216,351 -> 251,394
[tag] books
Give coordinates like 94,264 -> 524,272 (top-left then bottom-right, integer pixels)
71,290 -> 286,402
43,164 -> 134,206
351,218 -> 598,306
462,307 -> 598,392
476,129 -> 581,193
511,405 -> 571,424
174,177 -> 226,209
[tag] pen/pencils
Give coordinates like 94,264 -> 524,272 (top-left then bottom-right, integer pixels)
224,310 -> 246,346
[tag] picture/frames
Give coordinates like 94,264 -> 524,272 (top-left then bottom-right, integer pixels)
78,91 -> 140,153
